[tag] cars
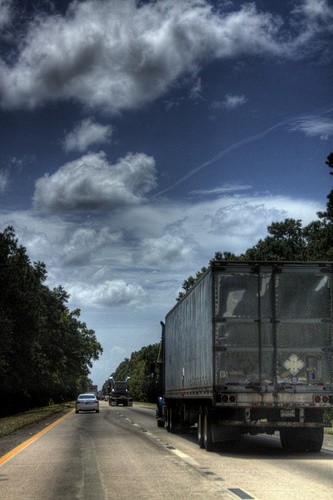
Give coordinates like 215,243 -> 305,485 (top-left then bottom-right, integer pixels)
74,393 -> 100,414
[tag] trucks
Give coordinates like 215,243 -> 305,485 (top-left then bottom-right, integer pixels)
107,376 -> 133,406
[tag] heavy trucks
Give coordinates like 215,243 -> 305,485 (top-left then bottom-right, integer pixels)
144,261 -> 333,454
